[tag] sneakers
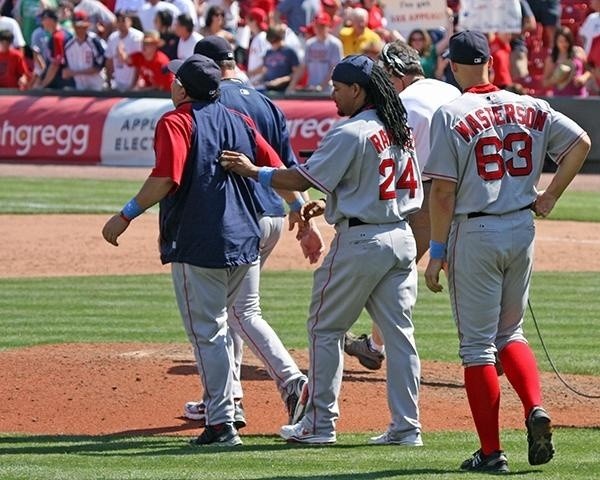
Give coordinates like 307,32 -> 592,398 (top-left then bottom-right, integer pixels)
459,448 -> 509,474
286,375 -> 308,425
368,429 -> 424,448
342,332 -> 386,370
526,407 -> 554,466
184,400 -> 246,448
279,419 -> 336,445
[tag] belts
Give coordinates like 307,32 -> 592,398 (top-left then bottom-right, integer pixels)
468,205 -> 535,219
349,217 -> 362,227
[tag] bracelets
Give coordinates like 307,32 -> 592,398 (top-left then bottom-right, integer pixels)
428,238 -> 446,259
122,198 -> 147,217
258,163 -> 278,188
288,196 -> 306,210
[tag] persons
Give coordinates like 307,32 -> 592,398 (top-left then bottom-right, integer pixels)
340,41 -> 506,377
0,0 -> 599,98
101,53 -> 312,450
425,30 -> 590,474
181,34 -> 320,422
217,55 -> 424,447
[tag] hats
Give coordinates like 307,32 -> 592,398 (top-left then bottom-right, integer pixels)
194,36 -> 235,60
144,29 -> 160,43
442,30 -> 491,65
168,54 -> 221,95
72,11 -> 89,28
35,9 -> 57,18
248,7 -> 269,31
315,12 -> 332,25
